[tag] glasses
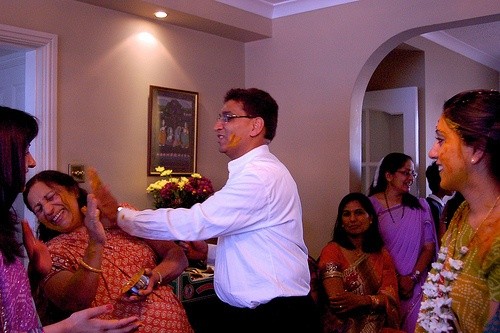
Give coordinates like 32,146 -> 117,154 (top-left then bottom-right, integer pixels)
216,113 -> 256,122
396,170 -> 417,179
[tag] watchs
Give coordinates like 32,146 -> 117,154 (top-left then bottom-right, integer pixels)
118,207 -> 123,211
22,170 -> 192,333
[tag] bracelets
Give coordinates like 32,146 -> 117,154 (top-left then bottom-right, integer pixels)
410,270 -> 420,283
153,268 -> 162,284
80,260 -> 102,272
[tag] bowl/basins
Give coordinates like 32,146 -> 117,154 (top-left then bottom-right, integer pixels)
121,271 -> 151,297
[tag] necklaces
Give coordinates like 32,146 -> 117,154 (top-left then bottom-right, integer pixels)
415,246 -> 469,333
456,194 -> 500,253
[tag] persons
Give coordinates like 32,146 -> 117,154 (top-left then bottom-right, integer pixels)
318,193 -> 400,333
426,161 -> 466,229
415,90 -> 499,331
88,88 -> 311,332
368,152 -> 435,333
1,104 -> 144,333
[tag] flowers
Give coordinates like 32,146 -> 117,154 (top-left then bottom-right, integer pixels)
146,165 -> 215,209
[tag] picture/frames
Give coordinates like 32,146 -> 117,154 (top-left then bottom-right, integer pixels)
147,85 -> 198,176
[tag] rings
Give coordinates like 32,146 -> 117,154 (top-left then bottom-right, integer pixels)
89,219 -> 98,225
339,306 -> 342,307
